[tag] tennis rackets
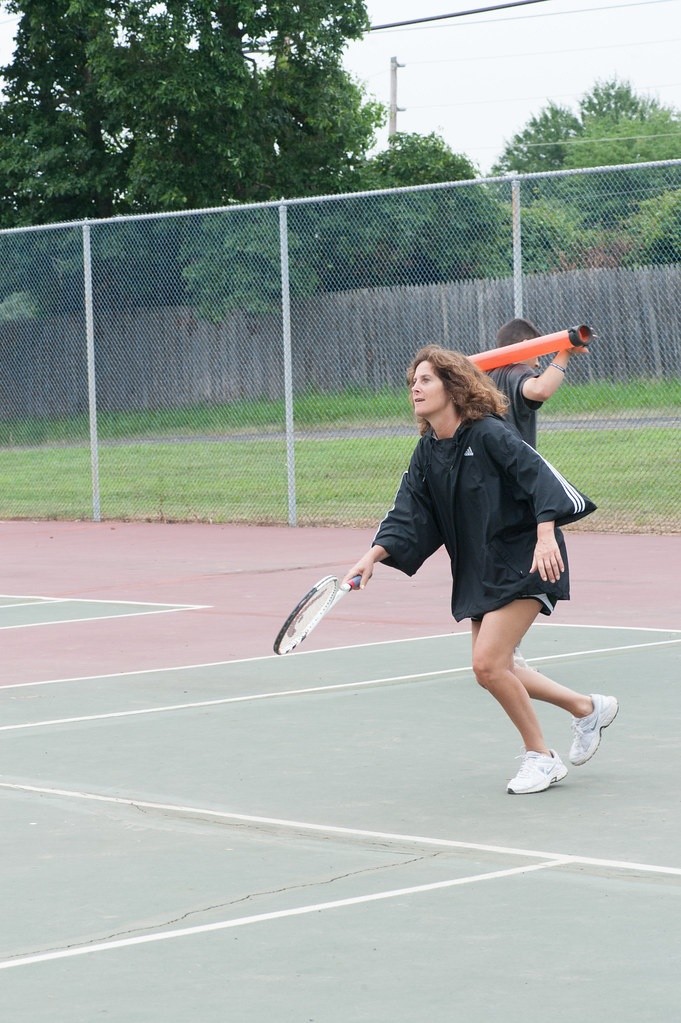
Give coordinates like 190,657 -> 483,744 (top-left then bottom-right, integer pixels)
273,572 -> 374,656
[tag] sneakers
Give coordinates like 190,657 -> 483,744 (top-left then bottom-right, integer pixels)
570,694 -> 619,766
507,749 -> 568,794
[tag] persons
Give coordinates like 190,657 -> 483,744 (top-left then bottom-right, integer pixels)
483,318 -> 589,673
342,343 -> 619,796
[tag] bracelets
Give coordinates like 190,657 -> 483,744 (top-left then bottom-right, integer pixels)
550,363 -> 565,373
